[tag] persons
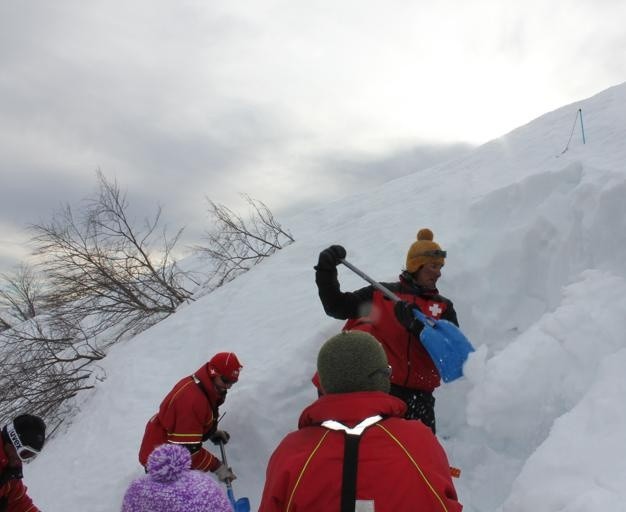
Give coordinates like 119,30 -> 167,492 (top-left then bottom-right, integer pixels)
313,227 -> 460,438
121,443 -> 235,512
138,351 -> 244,484
0,413 -> 46,512
256,328 -> 463,512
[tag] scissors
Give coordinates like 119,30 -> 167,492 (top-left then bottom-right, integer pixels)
338,256 -> 475,383
215,438 -> 250,512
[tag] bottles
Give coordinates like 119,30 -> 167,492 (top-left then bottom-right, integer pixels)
317,331 -> 391,393
121,443 -> 234,512
2,413 -> 45,461
208,352 -> 240,382
406,229 -> 444,273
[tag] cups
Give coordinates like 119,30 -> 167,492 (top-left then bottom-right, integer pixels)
409,250 -> 447,258
220,374 -> 237,384
5,417 -> 41,463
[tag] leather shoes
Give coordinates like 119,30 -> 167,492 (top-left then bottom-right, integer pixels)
395,299 -> 425,336
318,245 -> 346,269
208,430 -> 230,445
209,464 -> 237,482
